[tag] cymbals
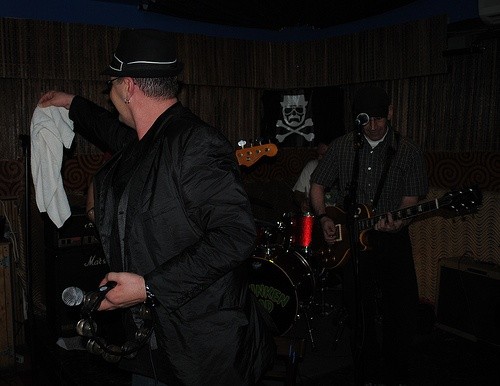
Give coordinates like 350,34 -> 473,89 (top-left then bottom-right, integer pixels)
76,281 -> 157,363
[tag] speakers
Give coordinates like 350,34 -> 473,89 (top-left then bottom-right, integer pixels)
434,256 -> 500,348
45,211 -> 112,341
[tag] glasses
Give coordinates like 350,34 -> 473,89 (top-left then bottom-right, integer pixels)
106,75 -> 137,89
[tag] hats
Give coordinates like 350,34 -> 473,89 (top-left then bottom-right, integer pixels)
351,87 -> 390,117
98,28 -> 183,78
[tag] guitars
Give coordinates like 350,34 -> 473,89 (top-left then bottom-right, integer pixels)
235,139 -> 278,168
312,182 -> 482,269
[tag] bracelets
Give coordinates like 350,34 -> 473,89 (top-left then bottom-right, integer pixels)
317,214 -> 326,221
145,283 -> 156,304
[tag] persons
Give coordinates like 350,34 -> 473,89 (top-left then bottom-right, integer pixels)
38,28 -> 276,386
310,86 -> 423,386
292,135 -> 335,213
86,152 -> 112,222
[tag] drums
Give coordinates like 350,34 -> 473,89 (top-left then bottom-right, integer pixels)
289,211 -> 316,254
246,243 -> 316,337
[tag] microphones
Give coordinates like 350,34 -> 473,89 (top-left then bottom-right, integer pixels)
59,286 -> 108,305
356,112 -> 370,126
